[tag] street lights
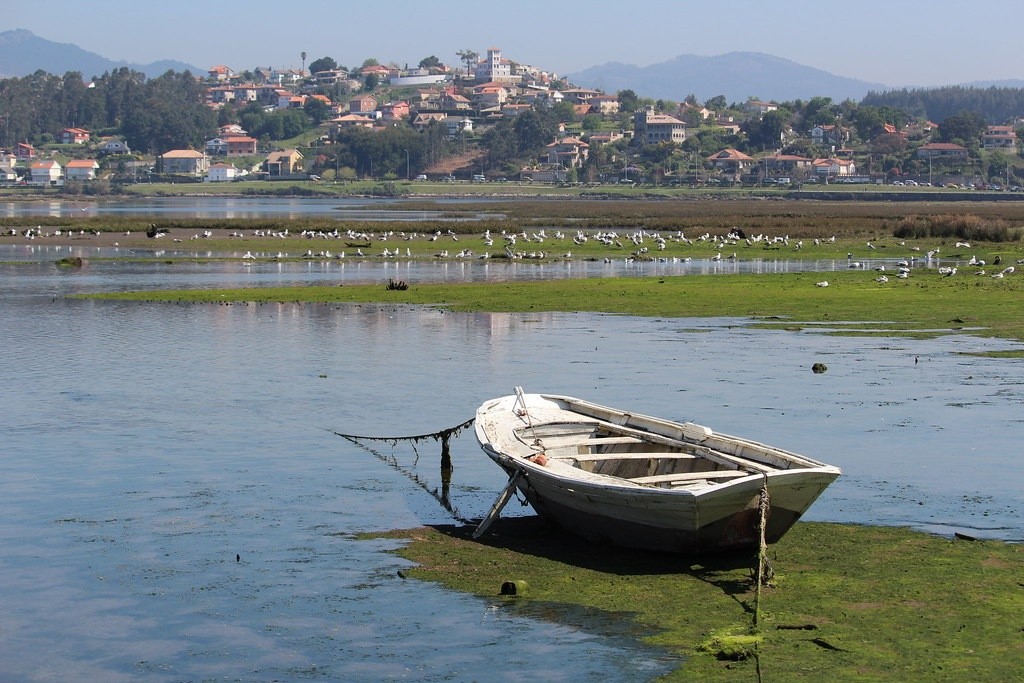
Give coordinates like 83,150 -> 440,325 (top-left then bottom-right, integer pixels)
404,149 -> 409,178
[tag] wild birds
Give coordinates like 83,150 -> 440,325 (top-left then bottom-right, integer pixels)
0,222 -> 1024,287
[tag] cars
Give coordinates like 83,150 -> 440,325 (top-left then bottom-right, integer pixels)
893,179 -> 1020,193
764,178 -> 777,183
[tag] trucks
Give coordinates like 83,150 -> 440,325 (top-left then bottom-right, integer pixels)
778,177 -> 790,183
416,174 -> 427,179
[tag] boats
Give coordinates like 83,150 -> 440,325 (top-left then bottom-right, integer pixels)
474,385 -> 844,558
